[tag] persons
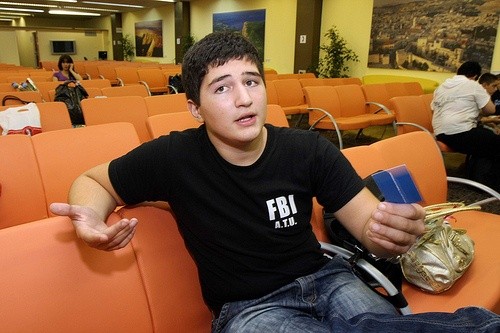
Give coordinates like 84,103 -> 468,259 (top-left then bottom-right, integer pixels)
488,74 -> 500,116
52,55 -> 84,82
47,31 -> 500,333
432,55 -> 500,191
472,72 -> 500,135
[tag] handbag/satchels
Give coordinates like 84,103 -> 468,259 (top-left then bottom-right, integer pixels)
399,201 -> 482,295
321,169 -> 402,285
55,80 -> 88,125
0,103 -> 42,136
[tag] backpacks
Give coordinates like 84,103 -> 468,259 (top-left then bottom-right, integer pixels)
169,73 -> 185,94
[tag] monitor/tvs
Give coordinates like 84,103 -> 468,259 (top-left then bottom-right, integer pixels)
50,39 -> 76,54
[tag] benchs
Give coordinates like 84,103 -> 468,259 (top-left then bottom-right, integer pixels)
0,58 -> 500,333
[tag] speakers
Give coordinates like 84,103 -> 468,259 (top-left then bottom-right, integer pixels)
99,51 -> 107,60
